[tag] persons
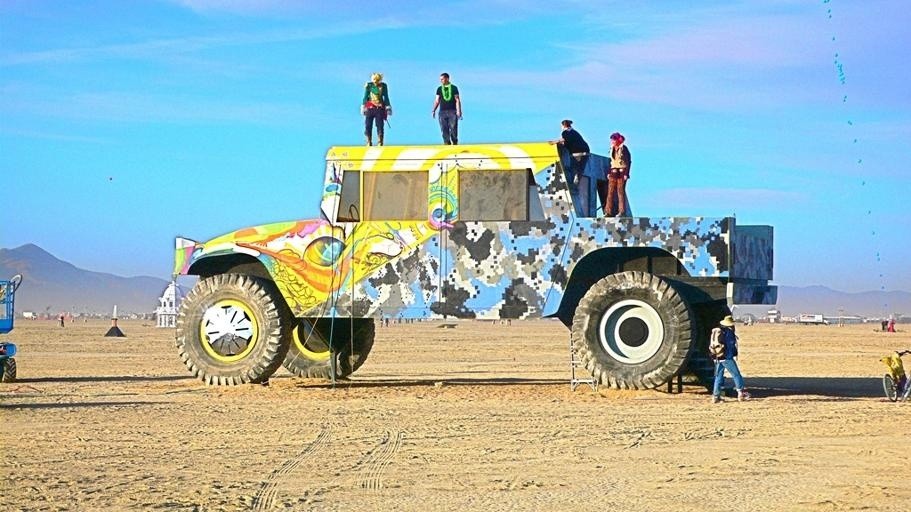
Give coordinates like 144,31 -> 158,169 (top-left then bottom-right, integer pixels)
882,316 -> 896,332
709,316 -> 751,404
602,132 -> 631,217
361,73 -> 392,146
547,120 -> 590,193
432,73 -> 462,145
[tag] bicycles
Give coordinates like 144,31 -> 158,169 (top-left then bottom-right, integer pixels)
879,350 -> 911,402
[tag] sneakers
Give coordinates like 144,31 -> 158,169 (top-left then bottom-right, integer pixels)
738,390 -> 751,402
600,213 -> 626,217
712,396 -> 725,404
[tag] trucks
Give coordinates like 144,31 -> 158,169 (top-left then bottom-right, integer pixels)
798,314 -> 832,326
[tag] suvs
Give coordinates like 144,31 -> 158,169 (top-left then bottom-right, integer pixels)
174,141 -> 777,390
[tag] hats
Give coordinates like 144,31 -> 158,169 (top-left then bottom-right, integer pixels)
720,315 -> 736,326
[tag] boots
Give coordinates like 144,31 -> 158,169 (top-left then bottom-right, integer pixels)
366,136 -> 383,146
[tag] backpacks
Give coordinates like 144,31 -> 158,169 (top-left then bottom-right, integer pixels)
709,328 -> 726,360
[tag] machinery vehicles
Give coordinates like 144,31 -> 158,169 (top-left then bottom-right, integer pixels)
0,274 -> 23,383
